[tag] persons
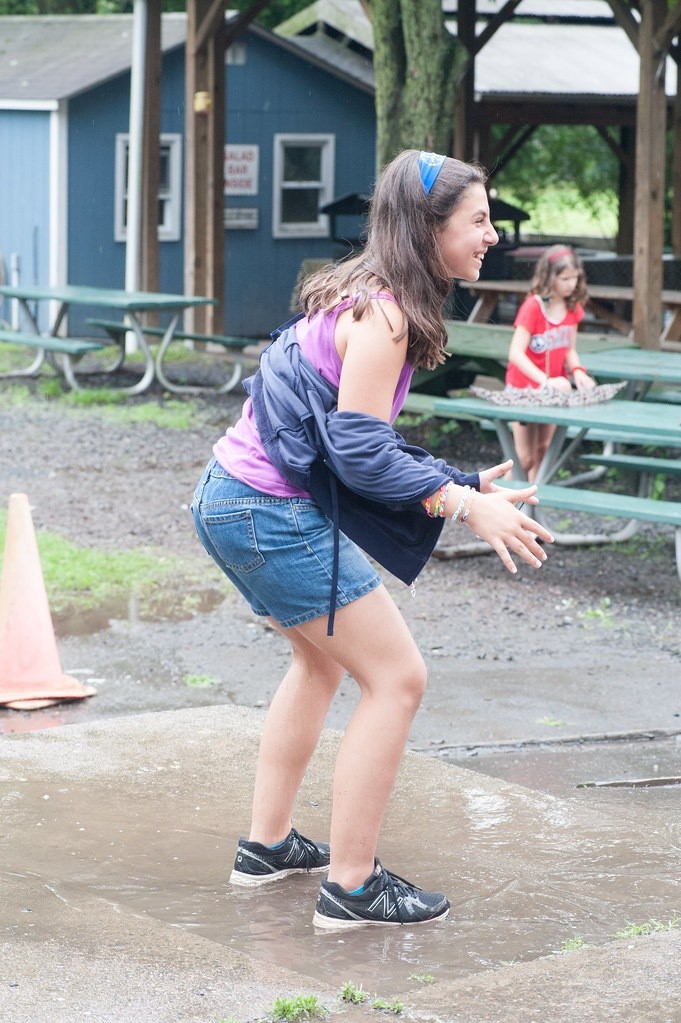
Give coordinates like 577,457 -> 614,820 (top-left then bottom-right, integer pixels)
188,147 -> 553,929
500,243 -> 596,482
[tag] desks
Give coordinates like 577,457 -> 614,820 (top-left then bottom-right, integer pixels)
402,281 -> 681,584
0,286 -> 245,397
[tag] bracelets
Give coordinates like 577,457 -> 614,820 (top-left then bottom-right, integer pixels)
424,480 -> 476,523
569,366 -> 587,375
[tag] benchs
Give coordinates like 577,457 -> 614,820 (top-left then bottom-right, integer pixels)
493,478 -> 681,528
88,318 -> 259,347
401,392 -> 481,423
481,419 -> 681,450
581,453 -> 681,477
0,330 -> 104,355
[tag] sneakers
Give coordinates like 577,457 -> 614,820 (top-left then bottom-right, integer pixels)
312,857 -> 451,928
229,827 -> 331,887
